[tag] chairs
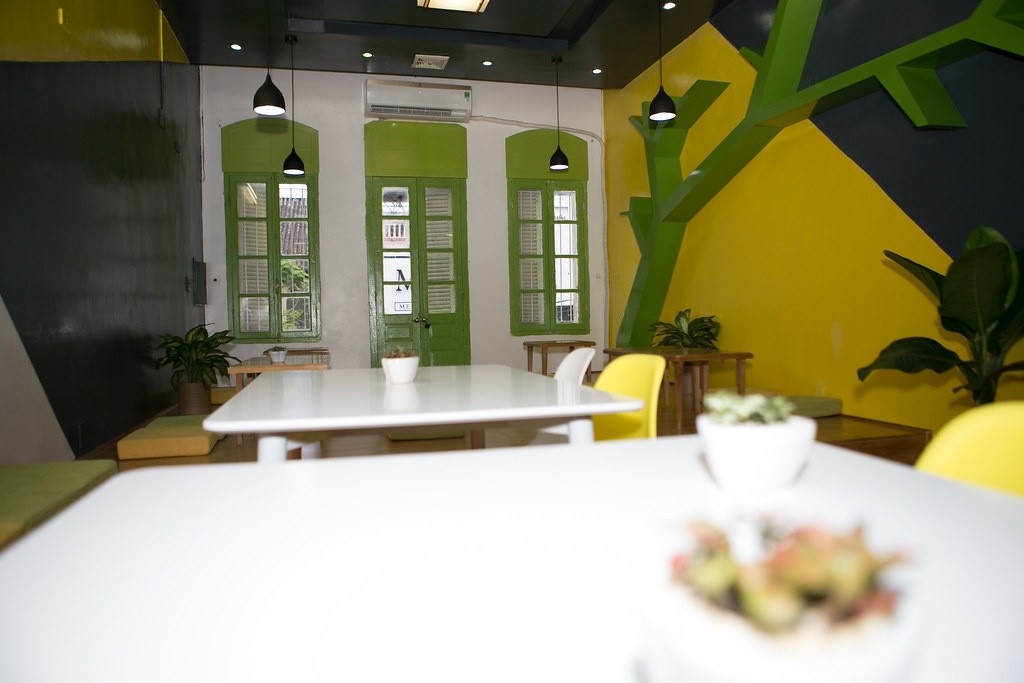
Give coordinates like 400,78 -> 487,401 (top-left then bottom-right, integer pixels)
510,347 -> 596,435
915,400 -> 1024,499
203,363 -> 647,462
586,355 -> 666,435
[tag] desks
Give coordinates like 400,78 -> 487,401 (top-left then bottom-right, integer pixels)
524,340 -> 596,383
263,347 -> 329,362
226,356 -> 329,449
805,413 -> 934,466
603,347 -> 712,409
630,349 -> 753,427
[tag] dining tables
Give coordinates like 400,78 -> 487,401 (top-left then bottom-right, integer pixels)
2,453 -> 117,547
4,428 -> 1022,683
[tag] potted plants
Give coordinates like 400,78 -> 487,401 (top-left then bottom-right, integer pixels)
379,346 -> 422,382
687,385 -> 819,485
859,225 -> 1022,406
649,308 -> 721,395
156,319 -> 243,417
266,344 -> 289,364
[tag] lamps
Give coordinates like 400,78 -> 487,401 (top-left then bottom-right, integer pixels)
251,71 -> 289,116
418,0 -> 490,15
281,146 -> 305,176
649,82 -> 678,121
550,143 -> 571,170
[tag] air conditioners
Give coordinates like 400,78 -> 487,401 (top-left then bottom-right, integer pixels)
364,78 -> 473,124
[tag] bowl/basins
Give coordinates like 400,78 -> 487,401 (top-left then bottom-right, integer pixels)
695,413 -> 820,496
268,350 -> 288,362
381,356 -> 420,383
